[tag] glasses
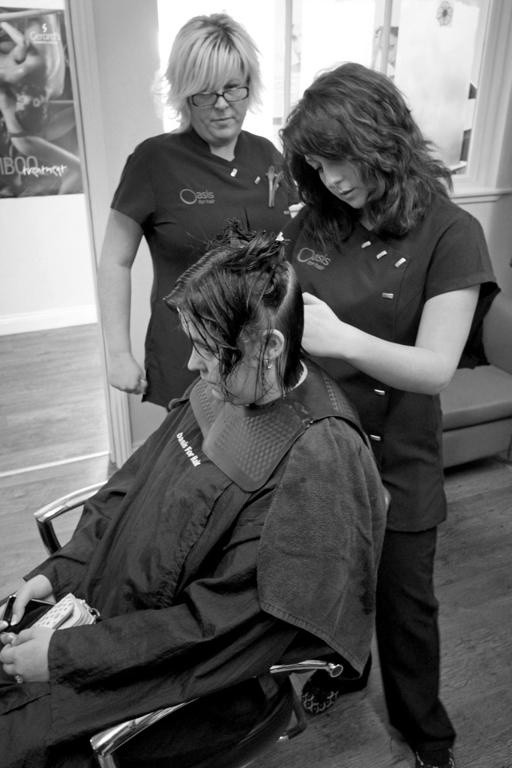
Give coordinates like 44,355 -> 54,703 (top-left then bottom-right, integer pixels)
188,83 -> 251,111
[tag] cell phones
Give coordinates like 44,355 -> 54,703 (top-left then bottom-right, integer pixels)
0,595 -> 57,634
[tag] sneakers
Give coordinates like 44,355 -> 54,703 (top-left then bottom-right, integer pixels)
298,656 -> 372,715
413,748 -> 455,767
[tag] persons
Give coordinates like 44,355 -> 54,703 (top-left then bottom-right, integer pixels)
1,9 -> 85,195
95,9 -> 303,413
275,60 -> 502,768
1,223 -> 394,768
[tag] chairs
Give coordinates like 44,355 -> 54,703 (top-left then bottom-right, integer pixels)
440,302 -> 512,474
32,379 -> 393,762
